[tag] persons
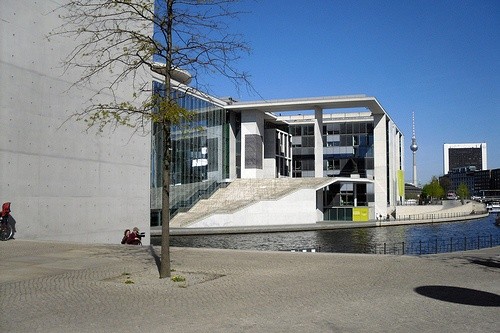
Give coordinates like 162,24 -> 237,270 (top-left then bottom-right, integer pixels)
127,227 -> 140,245
121,230 -> 130,244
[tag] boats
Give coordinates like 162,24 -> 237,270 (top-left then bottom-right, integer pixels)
469,196 -> 500,212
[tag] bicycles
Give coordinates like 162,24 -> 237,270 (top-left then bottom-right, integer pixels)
0,202 -> 14,240
